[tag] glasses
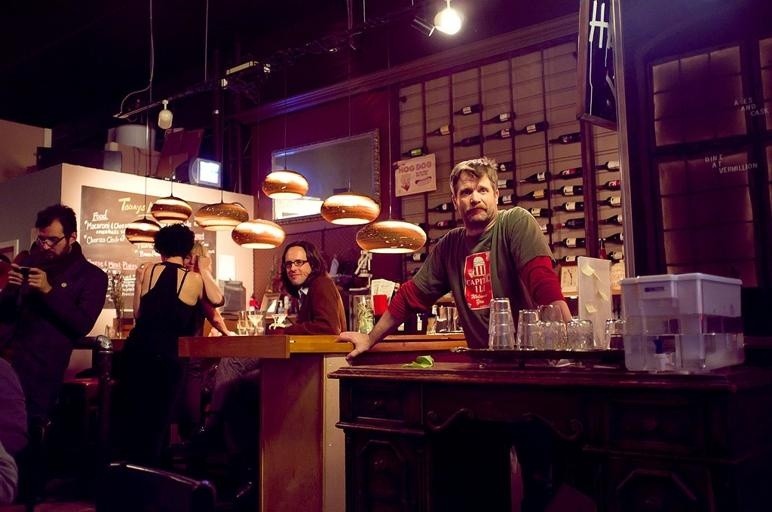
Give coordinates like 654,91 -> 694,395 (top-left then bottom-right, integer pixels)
282,259 -> 310,267
35,234 -> 69,247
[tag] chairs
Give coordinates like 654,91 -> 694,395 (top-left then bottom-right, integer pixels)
106,459 -> 254,512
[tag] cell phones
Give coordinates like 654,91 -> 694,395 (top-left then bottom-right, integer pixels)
12,267 -> 37,280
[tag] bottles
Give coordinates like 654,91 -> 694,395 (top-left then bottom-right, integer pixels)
454,104 -> 483,116
483,113 -> 517,125
452,136 -> 484,147
497,160 -> 625,267
400,146 -> 424,158
549,131 -> 582,145
517,121 -> 550,137
404,203 -> 457,276
486,127 -> 515,141
426,125 -> 454,137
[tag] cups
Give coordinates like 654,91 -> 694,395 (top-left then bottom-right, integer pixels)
237,310 -> 288,336
488,294 -> 623,353
352,295 -> 374,334
440,307 -> 457,332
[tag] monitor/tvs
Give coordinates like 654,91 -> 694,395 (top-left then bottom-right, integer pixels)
175,157 -> 222,188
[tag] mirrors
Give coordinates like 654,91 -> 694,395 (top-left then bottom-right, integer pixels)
271,126 -> 381,223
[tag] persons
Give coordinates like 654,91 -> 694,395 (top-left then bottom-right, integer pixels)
1,205 -> 108,428
113,223 -> 238,437
268,240 -> 348,335
1,359 -> 29,503
335,159 -> 572,361
182,242 -> 225,308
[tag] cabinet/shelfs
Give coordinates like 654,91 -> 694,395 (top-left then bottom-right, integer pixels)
329,369 -> 772,512
397,31 -> 624,281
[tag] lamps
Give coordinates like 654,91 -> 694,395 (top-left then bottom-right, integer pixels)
125,217 -> 161,245
321,192 -> 381,226
151,193 -> 193,224
188,156 -> 223,189
194,200 -> 248,231
231,217 -> 286,249
158,99 -> 175,130
355,216 -> 427,255
261,167 -> 310,199
409,0 -> 465,40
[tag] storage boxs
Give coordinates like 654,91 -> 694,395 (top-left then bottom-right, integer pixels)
621,272 -> 745,375
